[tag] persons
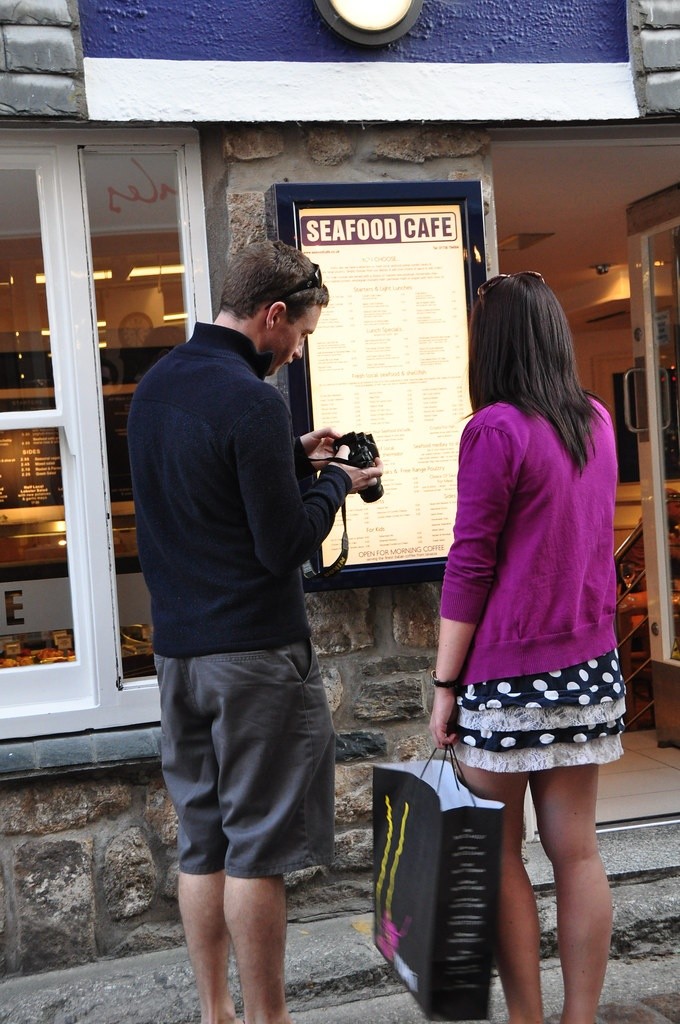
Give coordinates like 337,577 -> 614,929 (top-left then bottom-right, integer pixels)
128,239 -> 383,1023
429,271 -> 627,1024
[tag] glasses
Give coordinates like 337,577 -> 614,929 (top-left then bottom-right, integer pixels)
477,270 -> 546,303
264,262 -> 322,311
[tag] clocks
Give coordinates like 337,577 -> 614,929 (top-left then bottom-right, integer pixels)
118,311 -> 154,348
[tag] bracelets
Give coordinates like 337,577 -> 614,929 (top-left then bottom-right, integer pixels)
432,671 -> 459,688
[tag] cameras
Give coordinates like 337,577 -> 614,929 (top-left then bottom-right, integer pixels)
333,431 -> 384,504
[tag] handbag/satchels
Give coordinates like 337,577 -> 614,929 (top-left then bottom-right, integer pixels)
372,740 -> 507,1022
